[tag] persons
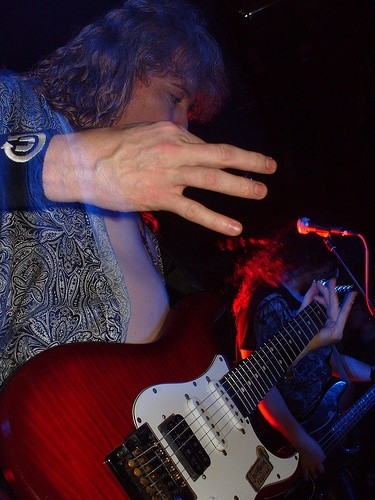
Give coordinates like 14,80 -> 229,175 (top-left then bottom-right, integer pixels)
0,1 -> 358,390
234,225 -> 375,480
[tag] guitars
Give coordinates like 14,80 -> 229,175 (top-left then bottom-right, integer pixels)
0,262 -> 356,500
256,381 -> 375,500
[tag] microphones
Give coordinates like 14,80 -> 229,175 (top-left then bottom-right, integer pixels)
298,217 -> 358,238
